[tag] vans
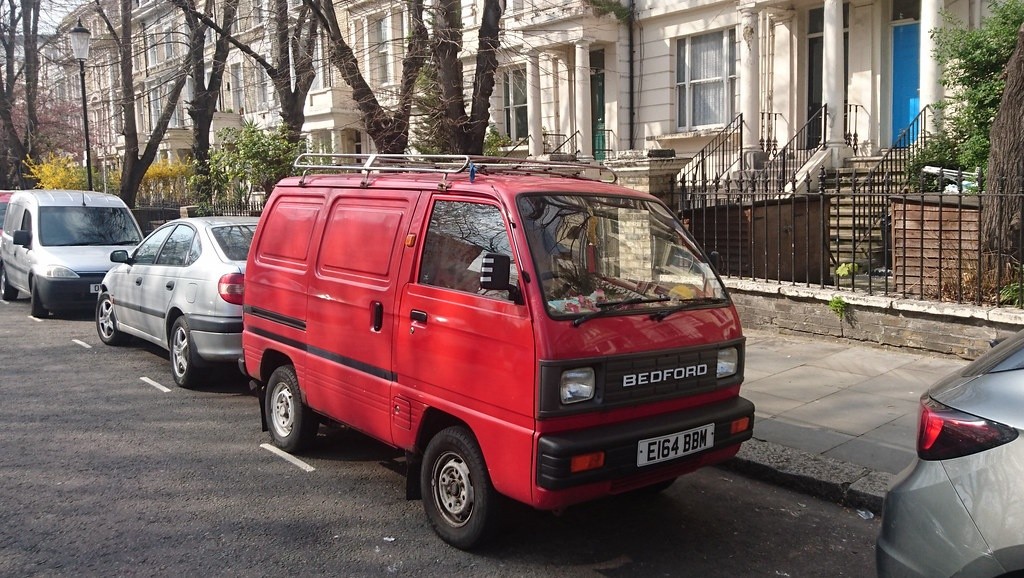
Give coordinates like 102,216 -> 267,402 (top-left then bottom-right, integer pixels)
0,187 -> 146,319
0,189 -> 18,236
240,151 -> 757,553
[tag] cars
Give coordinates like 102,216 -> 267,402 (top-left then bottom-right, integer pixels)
94,215 -> 261,389
874,326 -> 1023,578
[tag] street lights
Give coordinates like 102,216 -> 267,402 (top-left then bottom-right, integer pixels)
69,15 -> 94,192
